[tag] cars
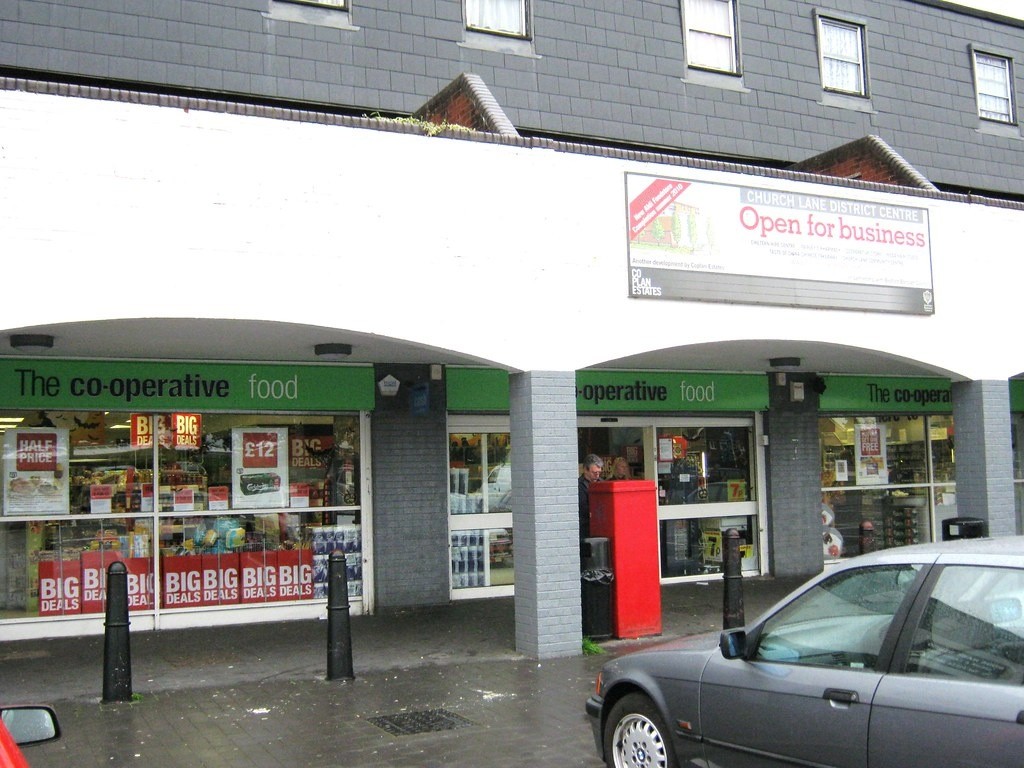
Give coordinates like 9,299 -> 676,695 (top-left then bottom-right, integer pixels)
585,532 -> 1024,767
684,467 -> 749,505
70,459 -> 206,517
451,492 -> 513,568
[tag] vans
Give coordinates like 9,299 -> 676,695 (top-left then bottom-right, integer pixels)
477,463 -> 511,494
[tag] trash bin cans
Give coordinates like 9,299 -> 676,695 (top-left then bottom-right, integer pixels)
579,536 -> 615,642
943,517 -> 987,541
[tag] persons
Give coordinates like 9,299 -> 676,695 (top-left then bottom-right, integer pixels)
577,453 -> 605,538
606,456 -> 635,480
